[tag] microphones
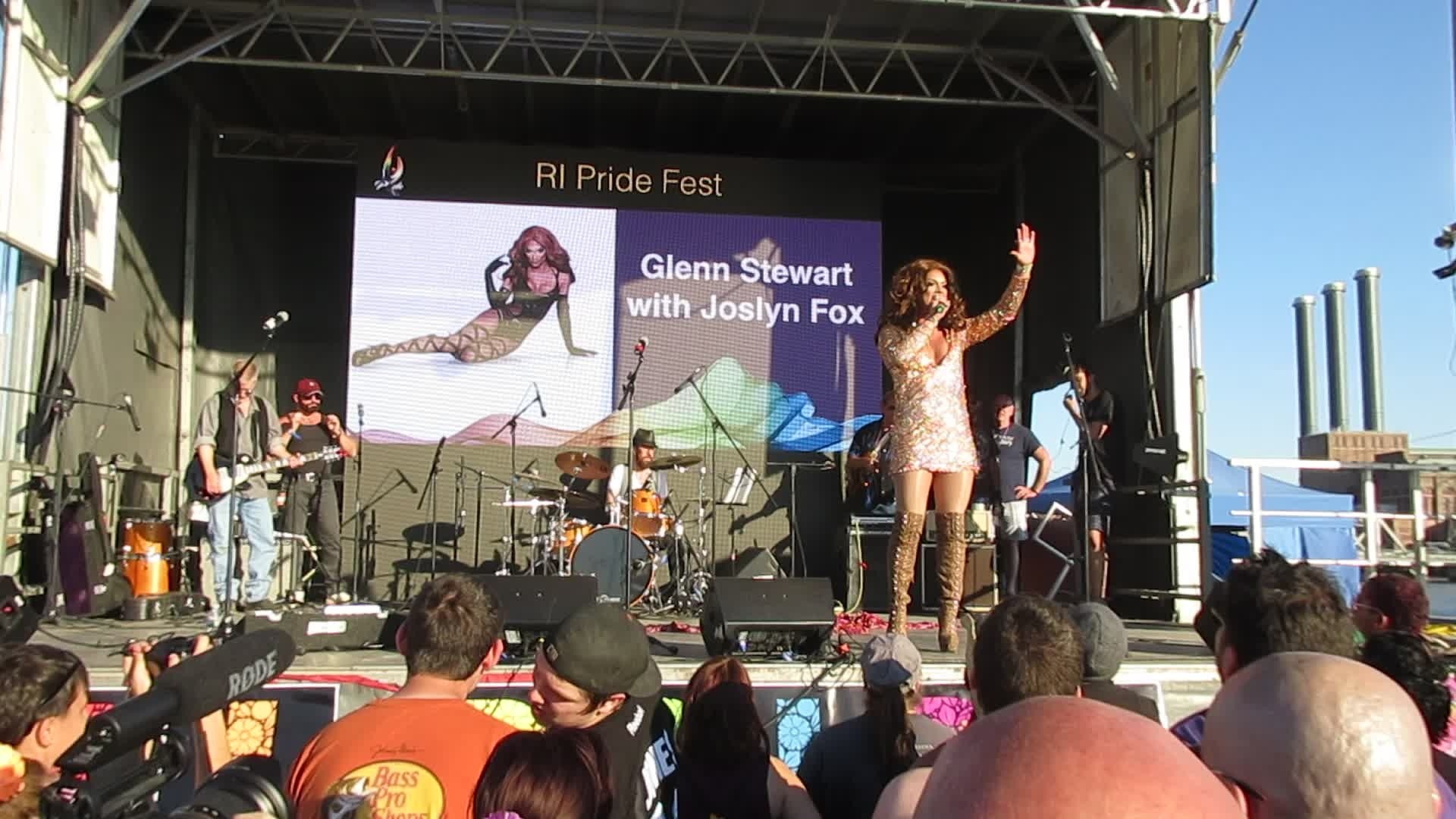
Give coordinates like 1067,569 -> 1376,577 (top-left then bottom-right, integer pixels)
357,403 -> 364,417
155,627 -> 296,725
263,311 -> 290,331
936,303 -> 946,315
122,393 -> 141,432
634,335 -> 649,355
674,368 -> 705,396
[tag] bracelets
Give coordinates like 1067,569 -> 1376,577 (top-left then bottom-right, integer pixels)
1030,488 -> 1039,496
1016,262 -> 1034,275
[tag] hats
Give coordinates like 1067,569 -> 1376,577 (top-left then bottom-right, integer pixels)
294,379 -> 324,396
630,428 -> 660,451
1071,599 -> 1129,679
862,633 -> 923,698
548,602 -> 664,697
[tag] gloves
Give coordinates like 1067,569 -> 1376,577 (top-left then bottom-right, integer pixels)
485,254 -> 510,308
557,293 -> 600,358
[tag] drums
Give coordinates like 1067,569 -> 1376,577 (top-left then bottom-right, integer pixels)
117,517 -> 177,597
568,525 -> 654,609
553,522 -> 595,555
619,488 -> 662,534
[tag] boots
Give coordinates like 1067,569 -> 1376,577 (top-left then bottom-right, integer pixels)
935,510 -> 966,652
888,506 -> 925,640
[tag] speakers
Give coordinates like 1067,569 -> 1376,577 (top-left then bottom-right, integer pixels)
458,574 -> 598,665
700,576 -> 834,661
0,592 -> 39,648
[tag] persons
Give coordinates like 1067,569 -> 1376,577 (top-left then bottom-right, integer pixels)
191,358 -> 305,618
0,544 -> 1456,819
352,224 -> 598,369
871,222 -> 1036,653
1063,361 -> 1124,605
970,391 -> 1052,598
265,378 -> 359,605
604,428 -> 686,604
845,389 -> 898,516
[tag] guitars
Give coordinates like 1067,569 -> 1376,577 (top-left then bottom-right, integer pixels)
182,444 -> 342,506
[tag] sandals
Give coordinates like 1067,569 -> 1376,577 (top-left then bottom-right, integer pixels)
353,323 -> 390,367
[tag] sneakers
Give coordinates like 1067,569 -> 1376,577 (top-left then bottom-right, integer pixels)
243,600 -> 282,614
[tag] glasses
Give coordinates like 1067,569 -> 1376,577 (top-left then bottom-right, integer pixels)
303,394 -> 322,400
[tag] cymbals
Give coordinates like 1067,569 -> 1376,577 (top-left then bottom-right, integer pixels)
554,451 -> 611,481
648,454 -> 703,470
527,487 -> 603,511
